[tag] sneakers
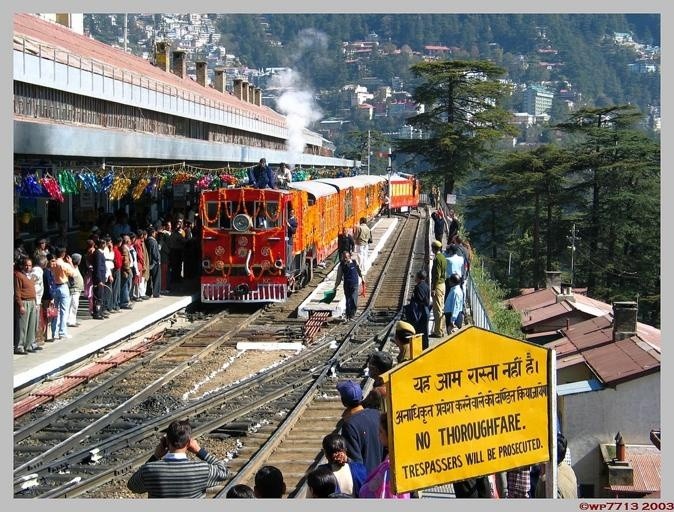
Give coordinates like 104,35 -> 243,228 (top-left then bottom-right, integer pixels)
13,295 -> 162,355
429,334 -> 444,338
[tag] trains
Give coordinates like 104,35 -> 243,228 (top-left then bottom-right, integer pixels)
192,171 -> 421,305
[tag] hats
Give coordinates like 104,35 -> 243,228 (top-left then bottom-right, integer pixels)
336,381 -> 362,400
369,351 -> 393,371
432,240 -> 442,247
395,320 -> 415,334
